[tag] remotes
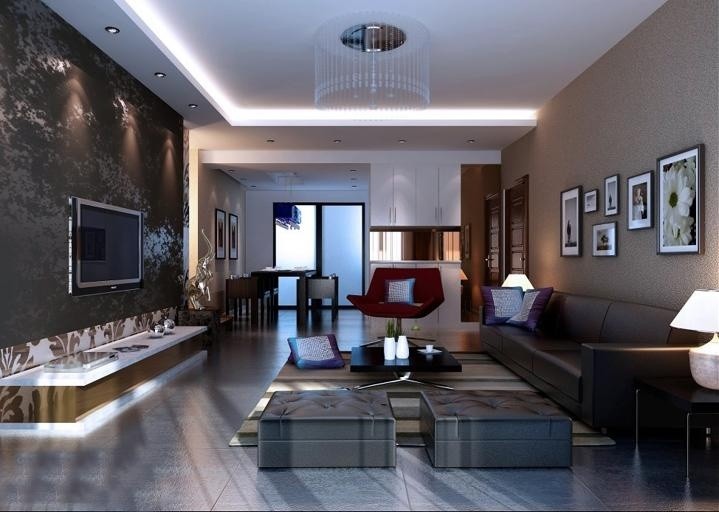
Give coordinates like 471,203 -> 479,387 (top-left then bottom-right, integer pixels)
132,344 -> 148,348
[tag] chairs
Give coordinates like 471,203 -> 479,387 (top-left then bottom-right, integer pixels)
347,268 -> 445,348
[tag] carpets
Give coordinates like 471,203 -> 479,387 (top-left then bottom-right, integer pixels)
229,352 -> 616,447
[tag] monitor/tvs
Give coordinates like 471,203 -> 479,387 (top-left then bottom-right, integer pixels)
68,195 -> 144,296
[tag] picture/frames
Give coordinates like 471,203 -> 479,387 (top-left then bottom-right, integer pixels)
584,189 -> 599,214
229,214 -> 239,260
604,174 -> 620,218
655,144 -> 705,256
627,170 -> 655,231
560,185 -> 583,257
592,221 -> 618,257
464,223 -> 472,260
215,208 -> 226,259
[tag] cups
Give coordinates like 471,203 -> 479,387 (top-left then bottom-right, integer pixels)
426,345 -> 433,352
265,266 -> 272,271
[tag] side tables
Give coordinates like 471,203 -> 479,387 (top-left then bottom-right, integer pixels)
178,306 -> 222,354
634,377 -> 719,478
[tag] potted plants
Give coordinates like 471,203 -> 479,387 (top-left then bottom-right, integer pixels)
383,319 -> 396,361
396,327 -> 409,360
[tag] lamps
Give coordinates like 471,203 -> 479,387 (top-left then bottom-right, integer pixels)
670,289 -> 719,390
460,268 -> 469,294
501,274 -> 535,293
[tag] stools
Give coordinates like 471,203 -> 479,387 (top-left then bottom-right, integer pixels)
297,277 -> 339,321
257,391 -> 396,467
225,278 -> 274,325
419,390 -> 572,468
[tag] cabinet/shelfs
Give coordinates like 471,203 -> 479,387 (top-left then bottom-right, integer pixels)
416,263 -> 461,330
416,164 -> 461,231
368,263 -> 416,332
370,163 -> 416,230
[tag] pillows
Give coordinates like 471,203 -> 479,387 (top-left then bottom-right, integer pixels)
384,277 -> 416,305
287,334 -> 345,369
505,287 -> 554,331
479,285 -> 523,326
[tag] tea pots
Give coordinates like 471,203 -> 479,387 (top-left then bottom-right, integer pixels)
158,316 -> 175,335
146,320 -> 167,338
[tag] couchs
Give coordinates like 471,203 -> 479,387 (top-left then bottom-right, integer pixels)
479,291 -> 714,435
